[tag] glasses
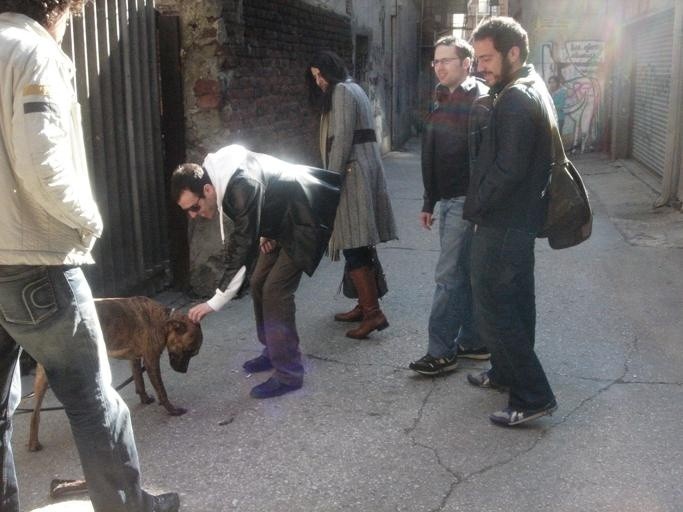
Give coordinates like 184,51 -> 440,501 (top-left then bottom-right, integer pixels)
182,197 -> 200,216
430,57 -> 459,68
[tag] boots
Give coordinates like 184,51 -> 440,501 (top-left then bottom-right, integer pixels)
334,305 -> 364,321
345,263 -> 389,338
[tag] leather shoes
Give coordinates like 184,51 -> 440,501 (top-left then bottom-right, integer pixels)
242,354 -> 272,373
250,374 -> 302,398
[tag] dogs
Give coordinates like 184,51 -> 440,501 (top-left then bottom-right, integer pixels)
28,296 -> 203,451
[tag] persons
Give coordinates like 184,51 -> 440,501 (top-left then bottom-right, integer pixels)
171,144 -> 340,398
461,16 -> 558,427
547,77 -> 567,136
1,1 -> 180,512
307,51 -> 399,339
410,36 -> 491,375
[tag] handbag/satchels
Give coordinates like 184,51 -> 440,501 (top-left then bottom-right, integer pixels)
342,259 -> 388,299
545,159 -> 593,249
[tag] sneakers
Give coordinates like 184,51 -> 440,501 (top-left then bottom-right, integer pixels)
408,352 -> 458,375
150,492 -> 178,511
467,366 -> 511,392
488,396 -> 558,426
455,337 -> 491,359
